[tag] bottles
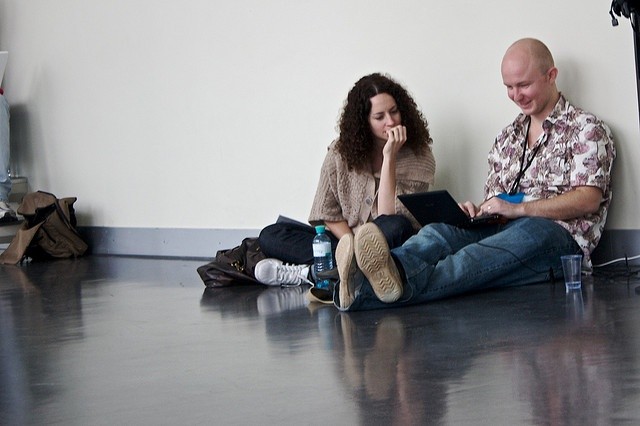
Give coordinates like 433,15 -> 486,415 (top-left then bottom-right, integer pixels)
312,225 -> 335,289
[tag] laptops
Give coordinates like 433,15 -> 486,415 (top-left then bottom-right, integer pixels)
398,190 -> 503,229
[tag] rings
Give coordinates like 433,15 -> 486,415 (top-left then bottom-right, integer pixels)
488,205 -> 491,210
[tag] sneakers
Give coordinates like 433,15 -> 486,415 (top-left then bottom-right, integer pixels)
335,233 -> 364,309
255,258 -> 315,291
353,221 -> 404,303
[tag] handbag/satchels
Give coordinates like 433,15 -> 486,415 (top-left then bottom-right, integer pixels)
197,237 -> 267,290
1,191 -> 90,264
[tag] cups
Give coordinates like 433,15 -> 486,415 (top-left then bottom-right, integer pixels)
560,255 -> 583,295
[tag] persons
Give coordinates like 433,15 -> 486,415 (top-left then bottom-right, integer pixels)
254,73 -> 436,287
333,38 -> 616,311
